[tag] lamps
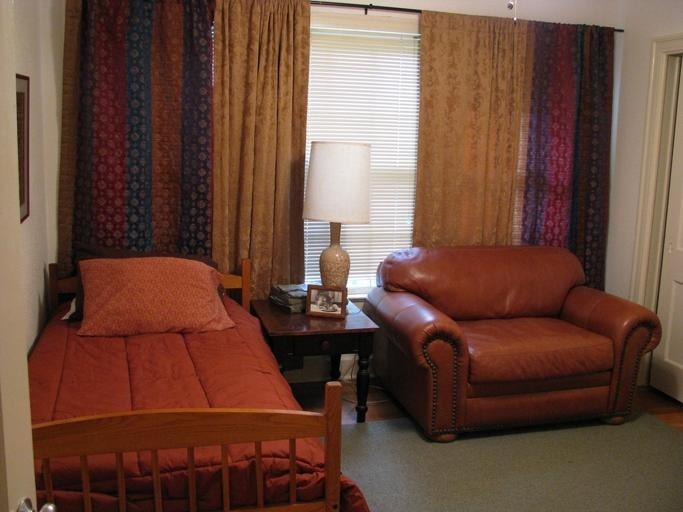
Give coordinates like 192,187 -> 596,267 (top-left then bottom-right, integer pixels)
302,140 -> 372,315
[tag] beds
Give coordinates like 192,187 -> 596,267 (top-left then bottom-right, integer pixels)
26,257 -> 342,511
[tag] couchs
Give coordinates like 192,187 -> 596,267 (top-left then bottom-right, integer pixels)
360,244 -> 662,444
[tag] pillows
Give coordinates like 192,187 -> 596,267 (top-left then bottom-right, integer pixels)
57,241 -> 236,337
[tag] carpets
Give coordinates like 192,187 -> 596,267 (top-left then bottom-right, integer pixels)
317,403 -> 683,511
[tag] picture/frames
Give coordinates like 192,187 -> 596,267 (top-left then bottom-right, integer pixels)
15,72 -> 29,223
304,284 -> 347,320
